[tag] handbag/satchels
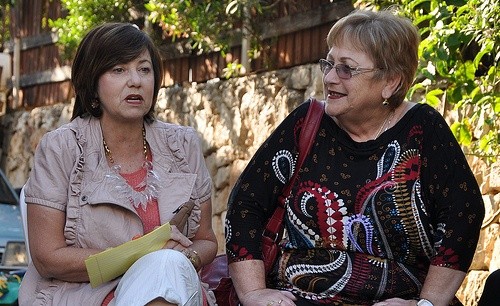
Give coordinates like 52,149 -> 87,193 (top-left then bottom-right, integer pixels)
213,234 -> 281,306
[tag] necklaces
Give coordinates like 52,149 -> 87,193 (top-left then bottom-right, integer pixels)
375,107 -> 394,141
104,120 -> 166,213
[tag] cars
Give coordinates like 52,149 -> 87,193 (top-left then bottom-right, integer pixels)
0,168 -> 28,278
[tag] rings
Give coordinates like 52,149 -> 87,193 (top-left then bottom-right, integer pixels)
279,299 -> 283,304
183,249 -> 190,257
267,301 -> 275,306
190,255 -> 197,263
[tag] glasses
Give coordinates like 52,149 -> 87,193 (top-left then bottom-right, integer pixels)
319,59 -> 383,79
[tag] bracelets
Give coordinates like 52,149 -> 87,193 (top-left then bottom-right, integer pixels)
189,247 -> 202,267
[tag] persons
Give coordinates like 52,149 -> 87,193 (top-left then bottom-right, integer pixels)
224,8 -> 484,306
17,23 -> 218,306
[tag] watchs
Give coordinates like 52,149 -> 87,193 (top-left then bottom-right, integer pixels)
416,298 -> 433,306
